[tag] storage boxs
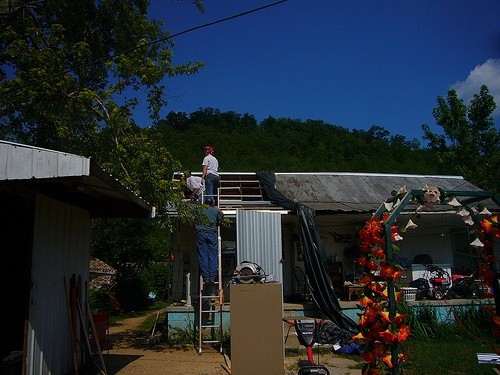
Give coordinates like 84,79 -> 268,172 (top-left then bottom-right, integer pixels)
400,286 -> 417,302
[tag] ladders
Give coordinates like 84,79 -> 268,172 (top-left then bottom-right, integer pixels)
198,187 -> 225,353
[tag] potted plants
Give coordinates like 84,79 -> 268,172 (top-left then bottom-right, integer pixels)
88,286 -> 118,349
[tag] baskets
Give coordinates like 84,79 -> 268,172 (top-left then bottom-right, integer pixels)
400,286 -> 418,301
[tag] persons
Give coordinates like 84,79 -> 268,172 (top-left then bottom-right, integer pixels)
202,147 -> 219,206
195,198 -> 224,283
180,170 -> 201,202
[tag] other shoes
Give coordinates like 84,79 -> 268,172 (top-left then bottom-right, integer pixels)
210,280 -> 214,285
205,280 -> 210,286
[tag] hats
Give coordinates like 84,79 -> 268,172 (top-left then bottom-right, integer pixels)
204,147 -> 214,154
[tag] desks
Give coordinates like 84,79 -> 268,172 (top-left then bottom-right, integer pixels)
345,283 -> 365,301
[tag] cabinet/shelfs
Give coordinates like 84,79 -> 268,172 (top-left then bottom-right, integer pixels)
327,262 -> 344,297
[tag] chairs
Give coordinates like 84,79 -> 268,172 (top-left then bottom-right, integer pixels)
293,266 -> 308,304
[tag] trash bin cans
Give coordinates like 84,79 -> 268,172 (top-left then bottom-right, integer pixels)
402,287 -> 418,302
